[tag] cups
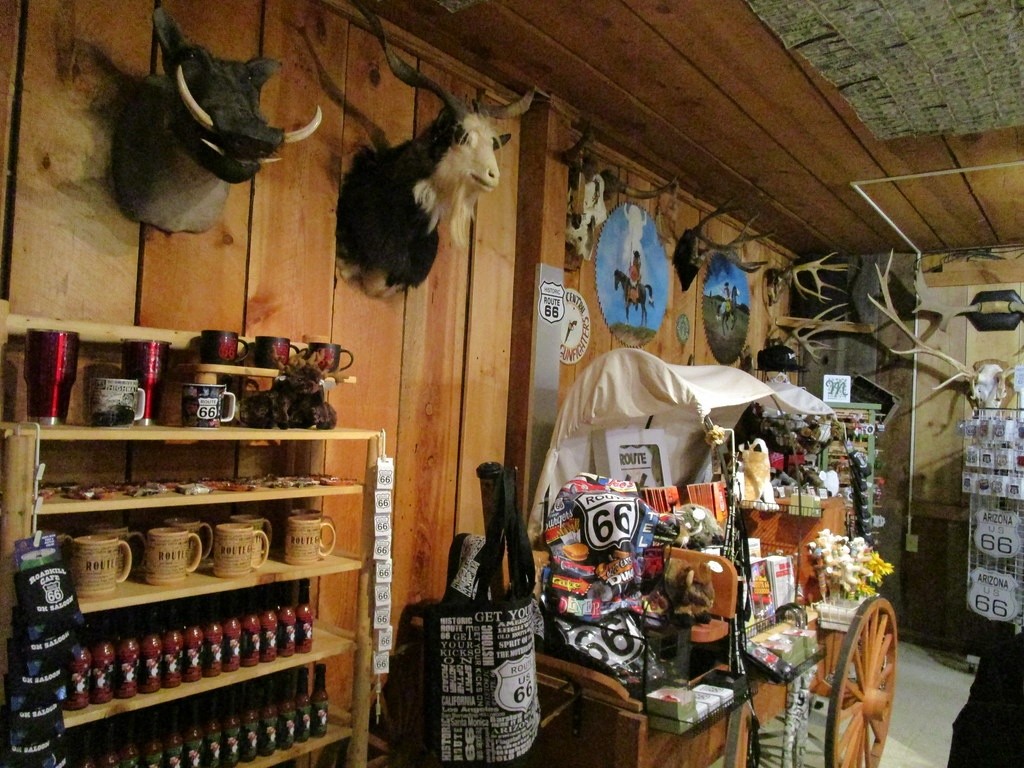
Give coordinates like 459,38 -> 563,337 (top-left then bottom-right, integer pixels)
41,508 -> 338,598
200,329 -> 249,364
89,377 -> 147,427
23,327 -> 80,425
181,383 -> 236,427
121,338 -> 173,426
308,342 -> 354,372
254,336 -> 300,369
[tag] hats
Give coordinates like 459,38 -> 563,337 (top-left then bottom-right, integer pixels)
757,346 -> 805,371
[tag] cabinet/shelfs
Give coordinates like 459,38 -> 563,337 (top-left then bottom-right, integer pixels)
1,301 -> 384,768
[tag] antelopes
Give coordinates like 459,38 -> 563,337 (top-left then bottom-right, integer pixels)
335,0 -> 537,297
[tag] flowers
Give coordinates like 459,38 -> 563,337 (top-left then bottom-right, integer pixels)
855,552 -> 895,596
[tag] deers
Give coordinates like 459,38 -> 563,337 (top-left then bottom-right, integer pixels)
672,197 -> 776,293
762,251 -> 854,364
867,248 -> 1023,418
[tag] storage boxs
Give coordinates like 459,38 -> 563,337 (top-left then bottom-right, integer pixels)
759,629 -> 818,667
591,427 -> 671,490
815,592 -> 881,633
647,687 -> 699,734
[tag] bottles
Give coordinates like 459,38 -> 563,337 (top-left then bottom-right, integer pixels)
78,663 -> 330,768
62,578 -> 314,712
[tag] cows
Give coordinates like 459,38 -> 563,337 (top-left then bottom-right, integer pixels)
559,118 -> 678,272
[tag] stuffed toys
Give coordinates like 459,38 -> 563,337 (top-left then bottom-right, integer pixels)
646,557 -> 715,627
676,503 -> 725,553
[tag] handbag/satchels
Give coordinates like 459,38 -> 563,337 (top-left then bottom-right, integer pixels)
423,468 -> 541,768
